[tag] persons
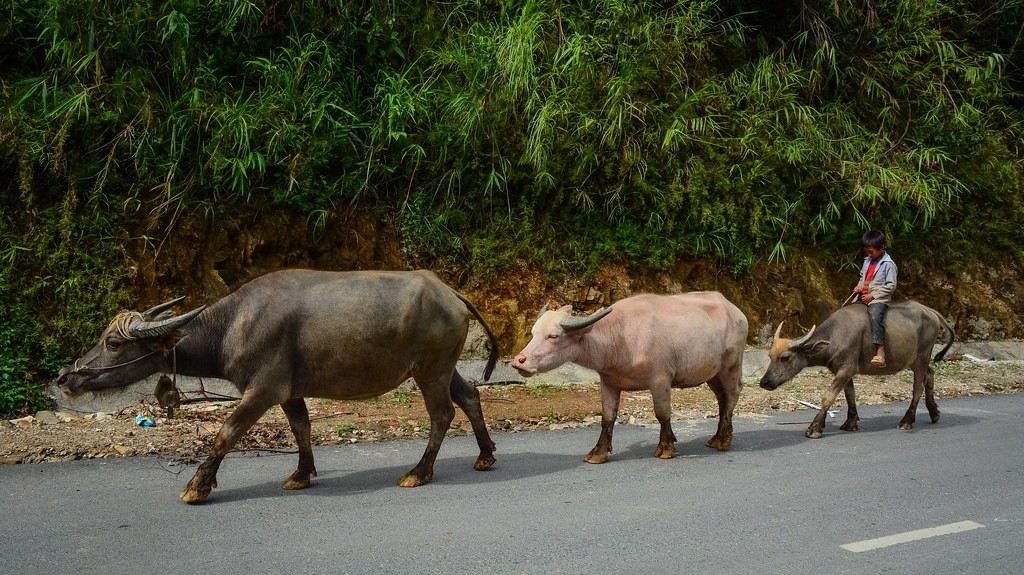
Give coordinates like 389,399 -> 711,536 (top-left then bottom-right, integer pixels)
848,228 -> 899,369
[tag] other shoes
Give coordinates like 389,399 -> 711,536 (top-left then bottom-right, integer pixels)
871,356 -> 886,368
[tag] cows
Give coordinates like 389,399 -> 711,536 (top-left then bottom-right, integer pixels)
759,297 -> 955,438
510,291 -> 749,464
57,269 -> 500,503
